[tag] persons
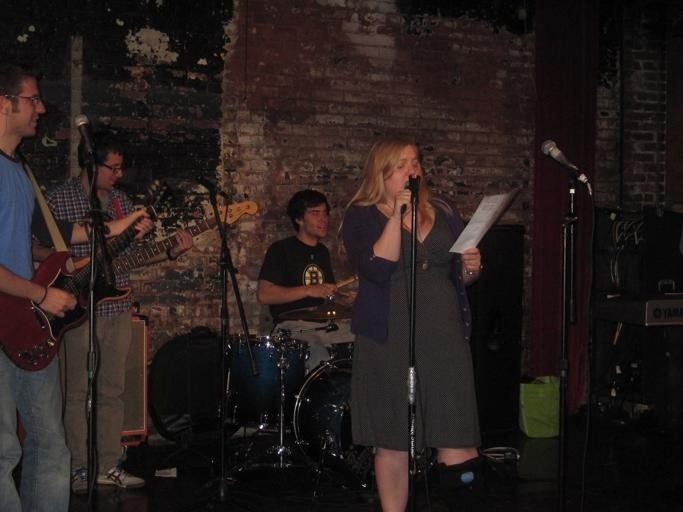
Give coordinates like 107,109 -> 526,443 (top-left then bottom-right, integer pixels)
256,190 -> 356,374
343,133 -> 481,510
0,61 -> 192,512
30,128 -> 193,494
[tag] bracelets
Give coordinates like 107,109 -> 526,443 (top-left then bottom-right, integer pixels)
32,285 -> 46,306
464,270 -> 480,275
167,247 -> 177,261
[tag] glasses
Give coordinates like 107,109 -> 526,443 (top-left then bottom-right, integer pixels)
5,93 -> 40,106
102,162 -> 122,177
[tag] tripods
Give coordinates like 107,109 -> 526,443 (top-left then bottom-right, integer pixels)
226,345 -> 340,485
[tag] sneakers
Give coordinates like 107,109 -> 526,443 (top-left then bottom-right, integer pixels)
96,468 -> 145,488
72,469 -> 88,493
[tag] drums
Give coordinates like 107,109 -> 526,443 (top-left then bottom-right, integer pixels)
224,333 -> 309,431
293,355 -> 438,494
327,341 -> 354,361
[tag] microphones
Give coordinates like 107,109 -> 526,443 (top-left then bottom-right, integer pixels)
74,113 -> 93,155
400,181 -> 410,212
540,140 -> 593,196
196,175 -> 227,198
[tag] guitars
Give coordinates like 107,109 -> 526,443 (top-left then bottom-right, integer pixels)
62,194 -> 258,308
0,179 -> 173,370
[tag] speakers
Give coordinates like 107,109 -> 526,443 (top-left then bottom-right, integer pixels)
466,224 -> 524,437
119,315 -> 149,447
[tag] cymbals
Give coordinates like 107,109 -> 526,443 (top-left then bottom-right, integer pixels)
287,302 -> 350,320
